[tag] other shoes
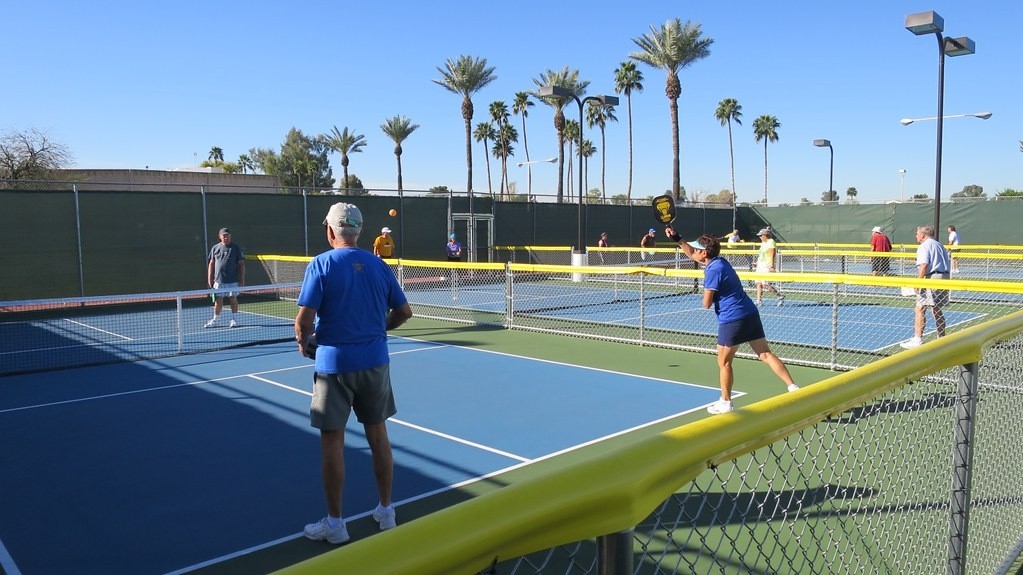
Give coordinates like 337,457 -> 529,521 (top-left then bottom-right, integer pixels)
777,296 -> 784,307
754,302 -> 763,307
952,269 -> 960,274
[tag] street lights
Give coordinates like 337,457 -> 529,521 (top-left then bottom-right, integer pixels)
813,139 -> 835,243
900,11 -> 994,242
517,156 -> 559,265
540,85 -> 620,255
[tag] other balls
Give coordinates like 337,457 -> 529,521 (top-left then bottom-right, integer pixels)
388,209 -> 398,217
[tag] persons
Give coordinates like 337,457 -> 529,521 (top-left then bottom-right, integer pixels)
294,202 -> 413,541
899,225 -> 950,350
598,233 -> 609,265
946,226 -> 960,274
751,229 -> 786,307
665,228 -> 801,415
372,227 -> 396,258
722,230 -> 746,263
203,227 -> 247,328
446,234 -> 465,286
641,228 -> 657,268
868,226 -> 894,288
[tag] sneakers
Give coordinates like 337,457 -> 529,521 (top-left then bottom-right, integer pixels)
707,403 -> 734,414
372,503 -> 397,530
304,517 -> 350,544
230,319 -> 238,328
204,320 -> 216,328
900,339 -> 922,350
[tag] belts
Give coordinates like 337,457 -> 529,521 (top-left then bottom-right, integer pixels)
928,271 -> 949,276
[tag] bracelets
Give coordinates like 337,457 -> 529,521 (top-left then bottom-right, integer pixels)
296,336 -> 308,344
771,267 -> 774,268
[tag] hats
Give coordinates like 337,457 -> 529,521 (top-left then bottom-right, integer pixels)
872,227 -> 883,234
382,227 -> 392,233
649,229 -> 656,233
322,202 -> 363,227
687,240 -> 706,250
755,228 -> 770,236
219,228 -> 230,235
451,234 -> 457,238
